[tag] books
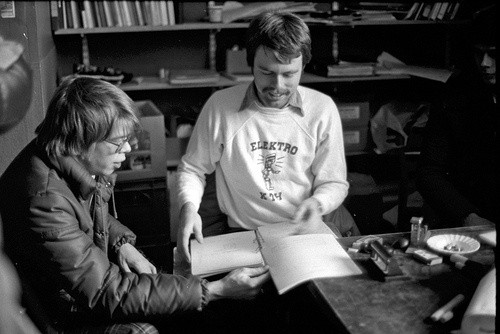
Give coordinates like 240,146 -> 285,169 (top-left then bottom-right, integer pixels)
405,1 -> 464,21
49,0 -> 175,30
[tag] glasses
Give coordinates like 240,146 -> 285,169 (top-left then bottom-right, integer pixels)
101,133 -> 131,154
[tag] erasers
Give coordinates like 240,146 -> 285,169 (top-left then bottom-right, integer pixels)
441,310 -> 453,320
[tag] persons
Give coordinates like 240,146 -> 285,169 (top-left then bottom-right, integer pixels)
425,4 -> 500,228
177,10 -> 349,263
0,74 -> 270,334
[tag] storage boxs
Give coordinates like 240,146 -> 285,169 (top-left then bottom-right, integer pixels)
117,100 -> 167,182
336,102 -> 369,154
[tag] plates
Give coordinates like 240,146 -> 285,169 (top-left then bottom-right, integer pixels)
426,234 -> 480,255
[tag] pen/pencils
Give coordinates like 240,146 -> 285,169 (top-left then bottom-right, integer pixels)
432,294 -> 464,321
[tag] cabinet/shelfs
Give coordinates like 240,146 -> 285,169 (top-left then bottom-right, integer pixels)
1,0 -> 461,248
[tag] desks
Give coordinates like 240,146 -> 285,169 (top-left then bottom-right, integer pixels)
307,223 -> 499,334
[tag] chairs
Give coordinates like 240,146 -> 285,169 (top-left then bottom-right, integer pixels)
199,173 -> 362,237
0,251 -> 71,334
